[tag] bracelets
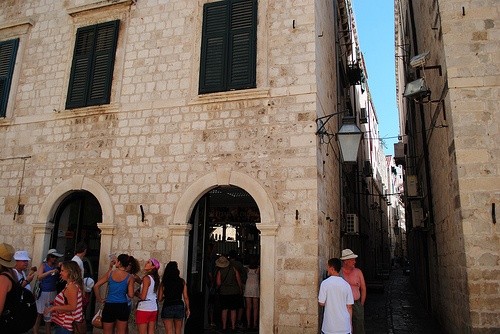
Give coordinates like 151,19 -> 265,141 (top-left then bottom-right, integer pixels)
25,280 -> 30,284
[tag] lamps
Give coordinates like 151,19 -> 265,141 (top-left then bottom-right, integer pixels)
316,110 -> 364,164
410,49 -> 430,88
404,78 -> 431,104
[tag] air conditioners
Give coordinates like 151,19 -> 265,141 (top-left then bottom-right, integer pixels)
347,214 -> 359,235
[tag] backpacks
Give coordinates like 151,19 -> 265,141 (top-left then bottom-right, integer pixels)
0,272 -> 38,334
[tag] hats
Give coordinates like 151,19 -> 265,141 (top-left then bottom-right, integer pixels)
339,249 -> 358,260
83,277 -> 94,292
149,258 -> 160,270
129,256 -> 140,274
13,251 -> 31,261
0,243 -> 17,268
47,249 -> 64,257
215,255 -> 230,269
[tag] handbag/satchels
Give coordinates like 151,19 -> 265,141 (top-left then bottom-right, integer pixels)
91,302 -> 103,329
72,318 -> 88,334
56,276 -> 66,291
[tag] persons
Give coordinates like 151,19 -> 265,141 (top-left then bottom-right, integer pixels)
316,258 -> 354,334
158,260 -> 190,334
337,249 -> 366,334
0,242 -> 38,334
31,243 -> 93,334
243,255 -> 260,329
94,254 -> 160,334
216,256 -> 242,332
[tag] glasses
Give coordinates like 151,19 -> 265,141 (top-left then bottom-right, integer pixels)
49,254 -> 59,259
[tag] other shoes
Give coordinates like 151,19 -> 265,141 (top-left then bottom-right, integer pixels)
208,320 -> 257,333
214,291 -> 220,307
